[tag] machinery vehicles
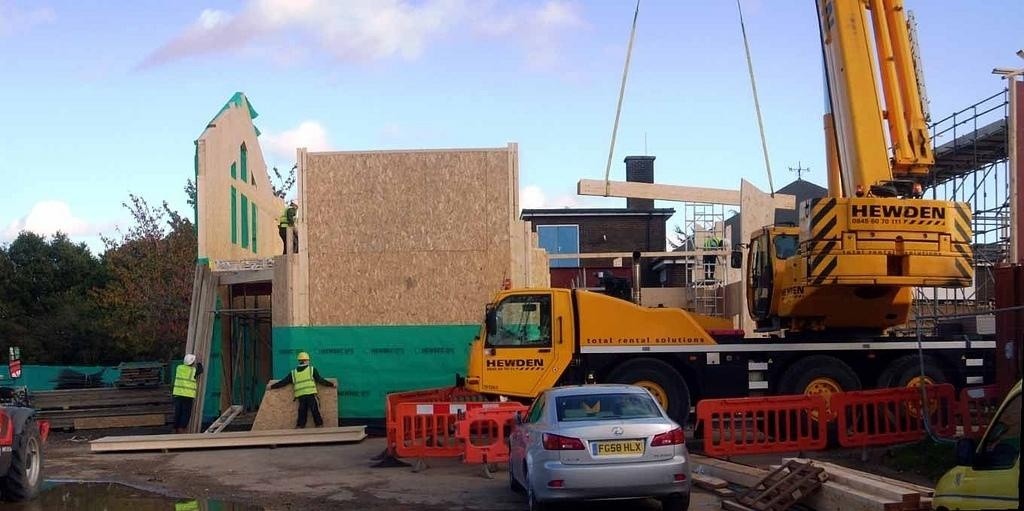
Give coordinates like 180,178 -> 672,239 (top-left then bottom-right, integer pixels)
456,0 -> 999,448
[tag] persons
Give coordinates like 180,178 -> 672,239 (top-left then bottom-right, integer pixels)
264,349 -> 338,430
701,237 -> 730,281
169,354 -> 204,435
276,198 -> 299,256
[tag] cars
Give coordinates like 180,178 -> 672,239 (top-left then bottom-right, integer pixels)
930,379 -> 1024,511
506,383 -> 691,511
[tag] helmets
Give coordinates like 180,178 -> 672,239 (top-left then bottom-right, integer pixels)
182,354 -> 196,366
291,198 -> 301,208
296,351 -> 310,362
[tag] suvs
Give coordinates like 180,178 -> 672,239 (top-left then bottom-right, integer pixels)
0,341 -> 49,502
